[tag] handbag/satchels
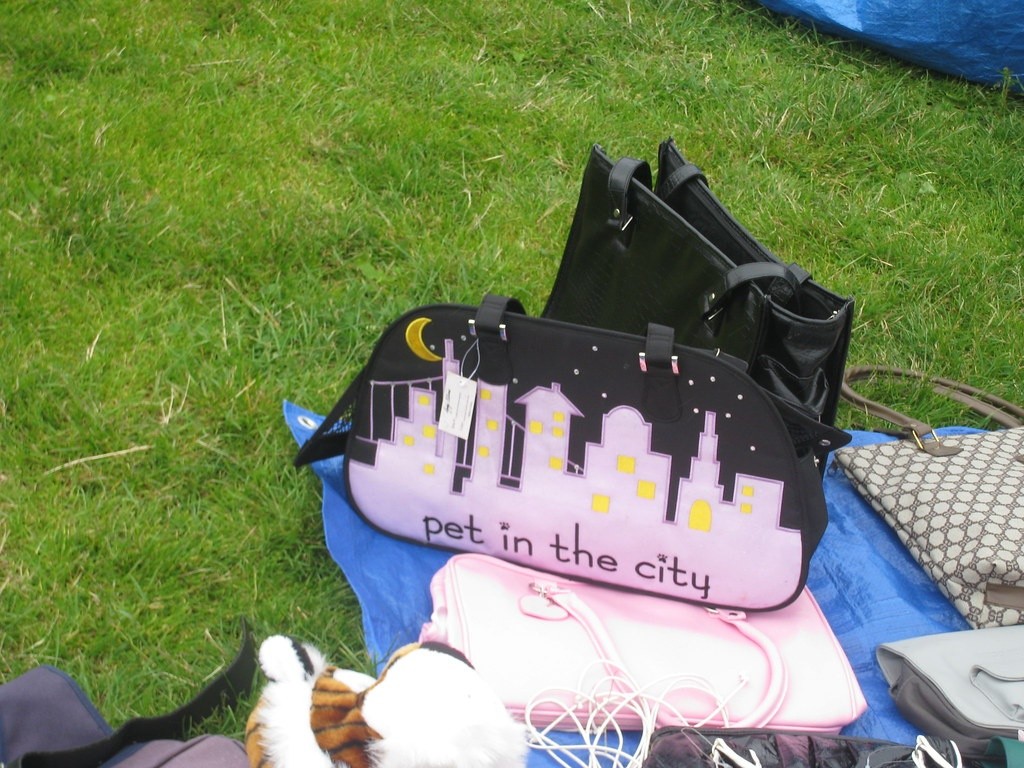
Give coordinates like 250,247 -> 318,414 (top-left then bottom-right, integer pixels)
299,307 -> 821,612
837,364 -> 1024,627
876,628 -> 1024,762
421,553 -> 868,737
536,138 -> 856,431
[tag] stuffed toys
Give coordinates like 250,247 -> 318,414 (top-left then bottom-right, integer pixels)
244,632 -> 529,768
